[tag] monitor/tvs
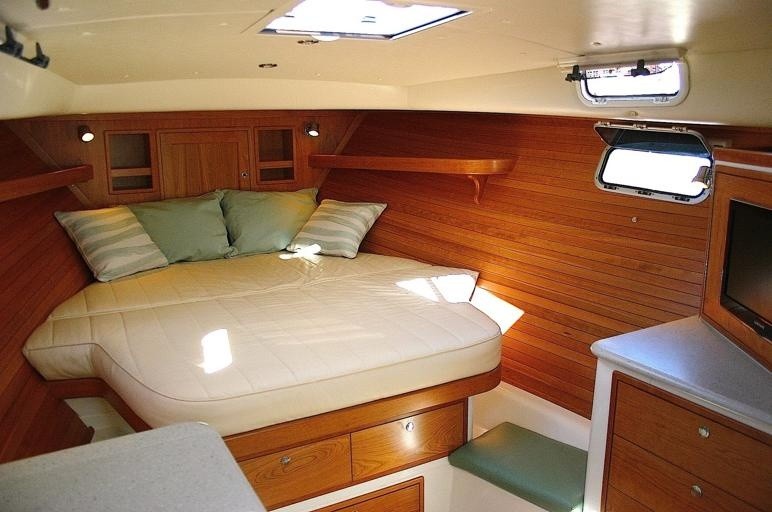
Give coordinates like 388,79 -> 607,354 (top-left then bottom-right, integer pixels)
720,199 -> 772,342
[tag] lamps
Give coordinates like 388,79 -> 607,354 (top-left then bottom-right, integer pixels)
299,118 -> 323,137
76,122 -> 96,145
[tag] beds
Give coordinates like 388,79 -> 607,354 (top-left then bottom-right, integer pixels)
17,183 -> 505,461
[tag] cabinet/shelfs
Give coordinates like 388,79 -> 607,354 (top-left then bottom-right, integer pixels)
158,130 -> 251,201
252,125 -> 301,184
600,370 -> 772,511
308,474 -> 424,511
236,400 -> 469,512
104,129 -> 158,193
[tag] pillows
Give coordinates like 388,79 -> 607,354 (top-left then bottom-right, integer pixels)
51,206 -> 171,283
128,190 -> 234,265
285,196 -> 388,261
216,186 -> 319,261
